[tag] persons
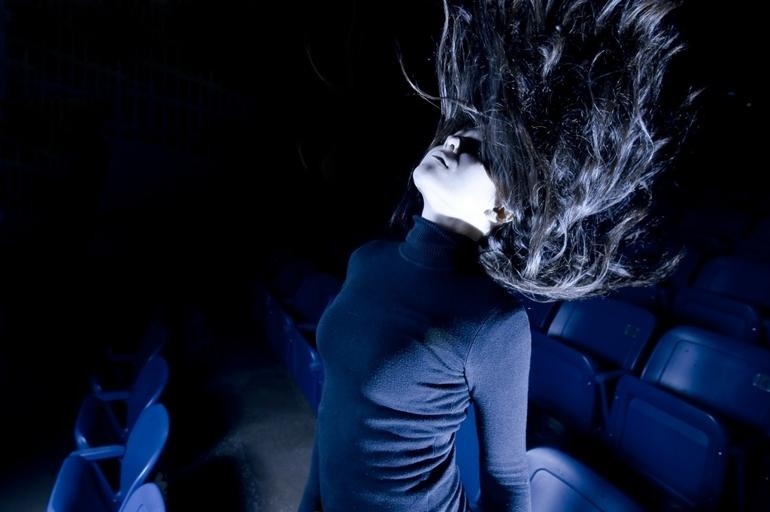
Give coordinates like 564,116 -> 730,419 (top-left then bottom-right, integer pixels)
294,1 -> 702,511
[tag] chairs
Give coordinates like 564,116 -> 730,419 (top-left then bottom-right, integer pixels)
45,353 -> 171,512
257,221 -> 770,512
